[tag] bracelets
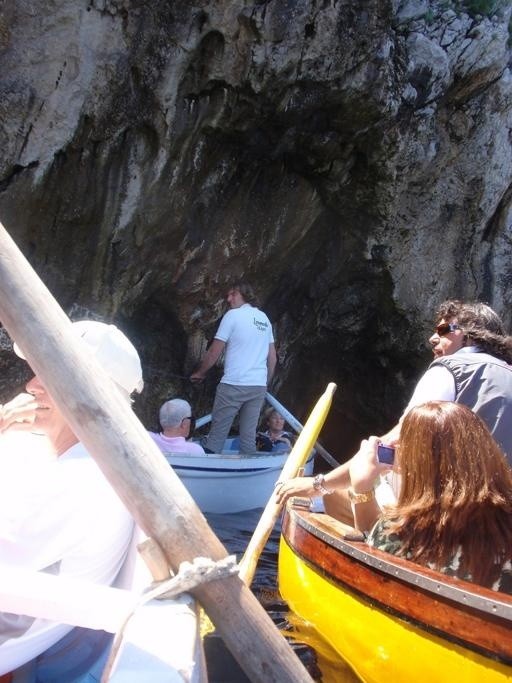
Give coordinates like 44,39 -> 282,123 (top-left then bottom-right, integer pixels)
314,474 -> 335,495
346,489 -> 375,504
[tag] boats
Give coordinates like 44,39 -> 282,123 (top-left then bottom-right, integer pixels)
277,485 -> 512,683
161,434 -> 316,514
2,515 -> 212,683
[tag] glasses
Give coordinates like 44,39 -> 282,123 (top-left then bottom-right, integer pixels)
437,322 -> 458,335
181,416 -> 197,423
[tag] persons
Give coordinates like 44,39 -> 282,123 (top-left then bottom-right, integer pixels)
272,298 -> 512,529
2,314 -> 148,683
262,406 -> 297,452
188,279 -> 279,457
347,400 -> 512,595
140,396 -> 206,458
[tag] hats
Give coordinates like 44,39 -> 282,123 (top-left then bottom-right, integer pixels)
12,320 -> 144,401
